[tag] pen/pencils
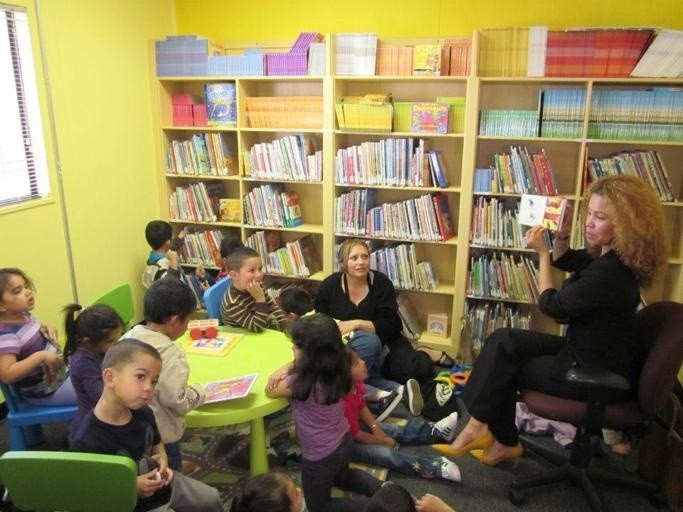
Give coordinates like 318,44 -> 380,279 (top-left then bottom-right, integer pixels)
38,353 -> 64,369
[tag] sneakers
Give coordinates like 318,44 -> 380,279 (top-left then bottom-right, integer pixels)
402,378 -> 425,417
374,390 -> 402,423
435,412 -> 458,441
440,455 -> 462,483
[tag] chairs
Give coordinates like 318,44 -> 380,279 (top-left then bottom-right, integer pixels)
85,283 -> 135,333
0,375 -> 138,512
511,302 -> 683,512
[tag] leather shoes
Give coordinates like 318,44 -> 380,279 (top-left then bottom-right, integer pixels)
416,346 -> 455,368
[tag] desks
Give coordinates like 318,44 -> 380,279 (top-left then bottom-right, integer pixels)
172,325 -> 296,476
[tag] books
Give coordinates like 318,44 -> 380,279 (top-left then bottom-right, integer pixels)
334,93 -> 466,135
587,150 -> 678,203
166,132 -> 323,228
175,225 -> 320,313
335,34 -> 471,76
465,250 -> 542,348
335,137 -> 450,188
333,238 -> 453,342
472,144 -> 561,196
171,83 -> 324,129
468,195 -> 556,250
335,188 -> 455,242
478,28 -> 681,78
155,30 -> 327,77
476,89 -> 682,143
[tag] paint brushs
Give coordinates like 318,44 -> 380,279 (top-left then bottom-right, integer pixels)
547,230 -> 554,253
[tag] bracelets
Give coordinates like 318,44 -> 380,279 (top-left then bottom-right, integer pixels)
555,236 -> 569,240
366,422 -> 378,430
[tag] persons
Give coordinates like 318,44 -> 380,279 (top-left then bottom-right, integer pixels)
330,346 -> 463,483
599,424 -> 632,457
264,313 -> 390,511
313,237 -> 456,385
68,339 -> 223,511
227,471 -> 305,511
432,173 -> 668,470
61,300 -> 125,451
109,277 -> 206,475
364,481 -> 456,511
0,267 -> 88,448
274,286 -> 425,423
139,220 -> 192,303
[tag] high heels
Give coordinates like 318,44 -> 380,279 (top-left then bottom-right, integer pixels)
469,442 -> 524,469
432,431 -> 494,464
